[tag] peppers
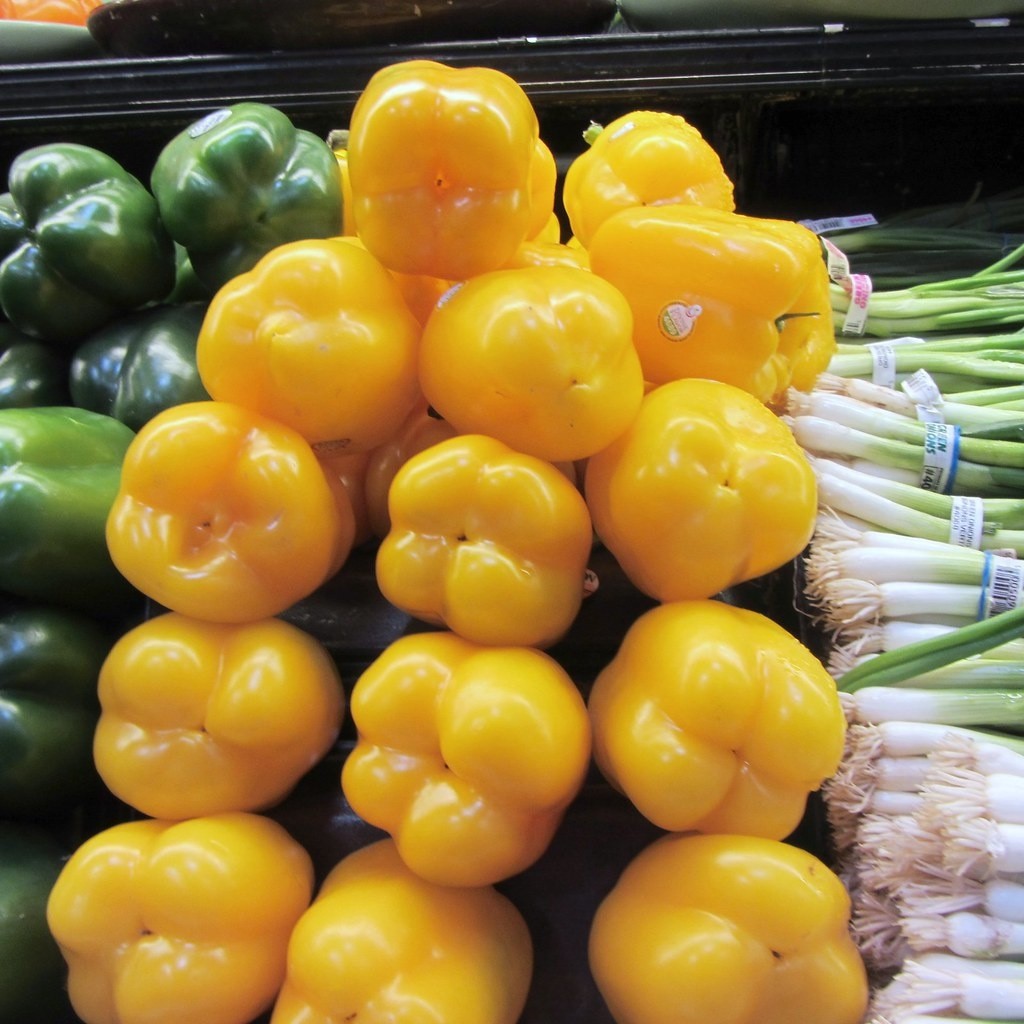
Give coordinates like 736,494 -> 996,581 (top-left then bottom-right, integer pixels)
0,61 -> 870,1024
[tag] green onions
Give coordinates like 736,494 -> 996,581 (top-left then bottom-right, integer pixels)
780,196 -> 1024,1024
